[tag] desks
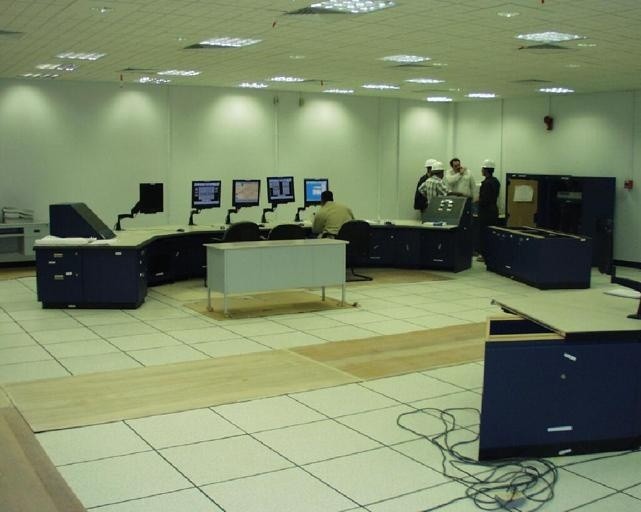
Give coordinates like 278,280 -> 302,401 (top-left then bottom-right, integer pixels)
200,239 -> 350,318
34,222 -> 473,310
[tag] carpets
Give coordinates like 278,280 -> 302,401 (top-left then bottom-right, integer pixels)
154,268 -> 451,302
182,290 -> 359,323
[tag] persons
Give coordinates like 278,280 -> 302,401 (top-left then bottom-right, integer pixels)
445,158 -> 474,200
418,161 -> 451,204
414,159 -> 438,222
476,160 -> 501,262
311,191 -> 354,239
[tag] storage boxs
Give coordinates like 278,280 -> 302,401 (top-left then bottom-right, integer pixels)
479,296 -> 641,461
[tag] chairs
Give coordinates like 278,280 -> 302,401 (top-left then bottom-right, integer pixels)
322,220 -> 371,281
205,221 -> 260,287
260,225 -> 305,240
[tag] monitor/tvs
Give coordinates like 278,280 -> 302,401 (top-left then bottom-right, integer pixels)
304,178 -> 328,207
232,180 -> 261,206
192,180 -> 221,209
266,176 -> 296,203
140,182 -> 163,213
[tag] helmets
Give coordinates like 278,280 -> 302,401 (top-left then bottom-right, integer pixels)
430,161 -> 444,172
480,159 -> 496,169
424,158 -> 437,168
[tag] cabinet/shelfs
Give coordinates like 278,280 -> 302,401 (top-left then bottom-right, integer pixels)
488,225 -> 592,292
0,222 -> 49,263
